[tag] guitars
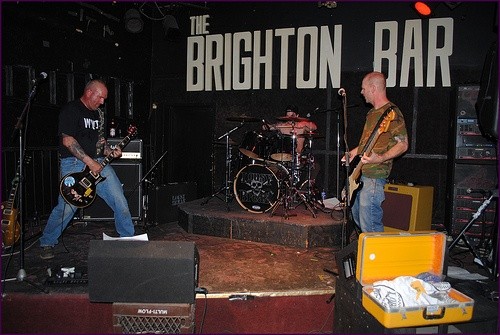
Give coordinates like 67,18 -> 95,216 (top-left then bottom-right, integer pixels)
0,153 -> 32,249
59,121 -> 138,208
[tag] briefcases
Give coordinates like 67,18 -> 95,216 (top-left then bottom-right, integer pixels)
356,230 -> 475,328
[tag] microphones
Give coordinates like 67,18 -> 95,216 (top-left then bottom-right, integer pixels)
337,87 -> 346,96
30,72 -> 47,93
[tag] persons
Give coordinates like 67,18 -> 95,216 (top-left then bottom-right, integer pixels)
341,72 -> 408,241
262,105 -> 320,205
40,79 -> 135,259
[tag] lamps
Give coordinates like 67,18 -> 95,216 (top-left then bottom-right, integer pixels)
123,9 -> 144,35
162,14 -> 179,40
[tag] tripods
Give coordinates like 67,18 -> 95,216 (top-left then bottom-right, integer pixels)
0,82 -> 56,294
447,140 -> 500,299
202,123 -> 332,220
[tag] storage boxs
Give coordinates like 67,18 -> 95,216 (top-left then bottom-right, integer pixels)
106,138 -> 143,160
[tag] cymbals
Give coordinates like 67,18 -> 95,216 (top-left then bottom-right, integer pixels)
226,117 -> 262,122
297,133 -> 326,139
276,117 -> 301,122
213,141 -> 240,146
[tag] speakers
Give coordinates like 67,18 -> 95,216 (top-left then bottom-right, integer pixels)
77,159 -> 142,220
148,182 -> 198,226
448,82 -> 500,259
400,155 -> 450,230
87,240 -> 199,303
474,32 -> 500,139
381,182 -> 433,233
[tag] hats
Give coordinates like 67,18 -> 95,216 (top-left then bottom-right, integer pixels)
285,104 -> 299,115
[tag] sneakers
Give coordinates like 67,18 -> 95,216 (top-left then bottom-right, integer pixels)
40,246 -> 55,259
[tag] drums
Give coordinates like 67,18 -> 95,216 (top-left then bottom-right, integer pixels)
233,160 -> 292,214
268,135 -> 298,162
237,130 -> 269,159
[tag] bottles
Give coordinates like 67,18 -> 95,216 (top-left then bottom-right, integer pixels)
109,118 -> 116,138
321,188 -> 326,199
